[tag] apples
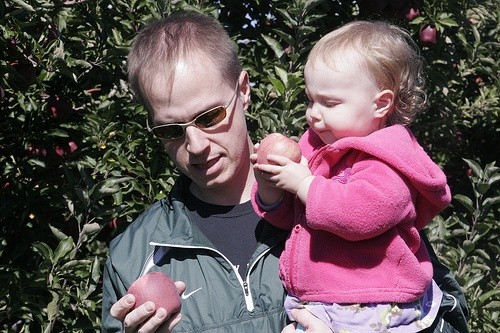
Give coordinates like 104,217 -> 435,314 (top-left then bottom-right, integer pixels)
254,133 -> 302,172
126,272 -> 182,325
406,2 -> 484,177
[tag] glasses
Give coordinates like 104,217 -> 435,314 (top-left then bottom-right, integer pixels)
146,79 -> 239,140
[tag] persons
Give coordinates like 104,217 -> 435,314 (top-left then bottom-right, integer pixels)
99,12 -> 473,333
248,19 -> 453,333
